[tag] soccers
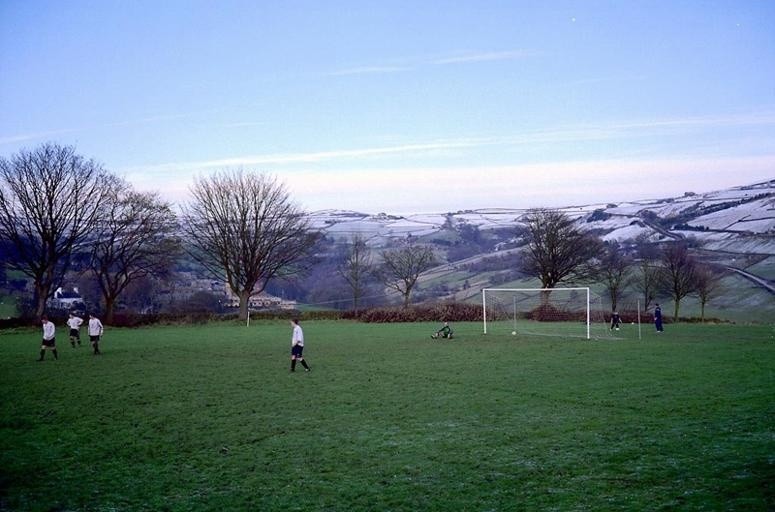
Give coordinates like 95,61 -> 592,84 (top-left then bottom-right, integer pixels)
512,331 -> 517,335
616,328 -> 619,330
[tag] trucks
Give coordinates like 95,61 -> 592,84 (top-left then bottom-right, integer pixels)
18,279 -> 85,318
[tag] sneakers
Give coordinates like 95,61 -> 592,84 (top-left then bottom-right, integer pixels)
430,335 -> 452,339
288,367 -> 311,372
36,346 -> 102,361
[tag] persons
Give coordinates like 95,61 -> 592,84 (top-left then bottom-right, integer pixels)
431,320 -> 455,340
654,303 -> 664,333
88,311 -> 104,354
290,317 -> 310,373
35,313 -> 58,362
609,310 -> 622,332
66,313 -> 84,349
